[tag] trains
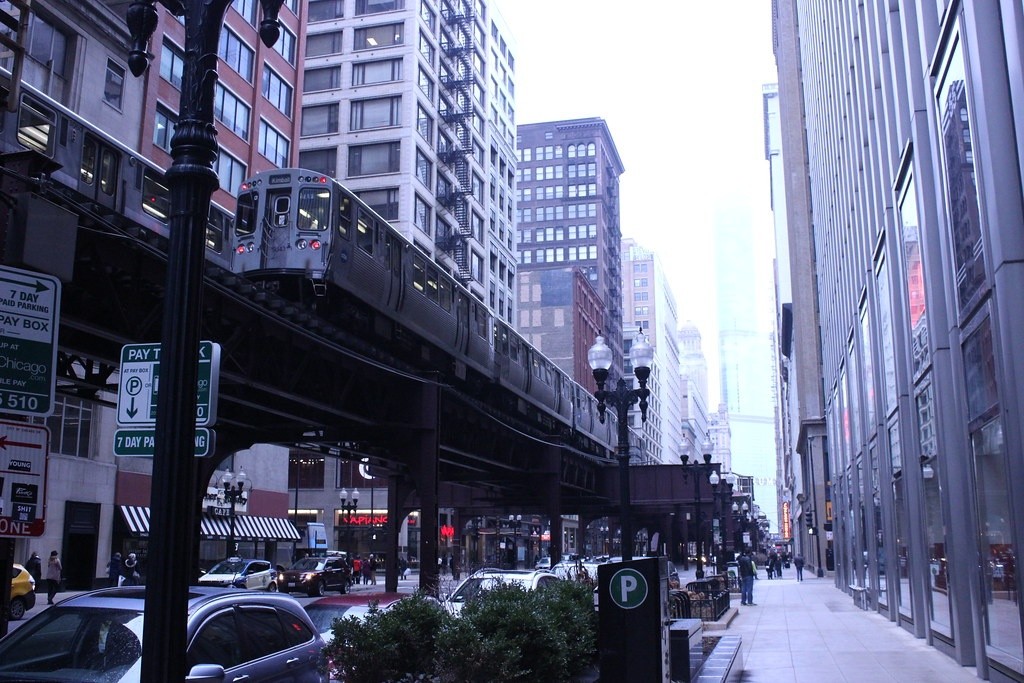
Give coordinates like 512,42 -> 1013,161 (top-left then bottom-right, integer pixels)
0,66 -> 238,284
232,164 -> 649,473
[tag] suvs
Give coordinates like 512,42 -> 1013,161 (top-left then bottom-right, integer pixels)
0,586 -> 330,683
279,555 -> 353,598
7,562 -> 37,620
197,555 -> 279,591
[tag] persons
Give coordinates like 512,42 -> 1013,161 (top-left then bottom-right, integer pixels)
991,548 -> 1015,607
25,551 -> 42,591
398,552 -> 462,581
107,553 -> 124,586
293,551 -> 378,585
738,548 -> 805,606
46,551 -> 62,605
123,553 -> 142,586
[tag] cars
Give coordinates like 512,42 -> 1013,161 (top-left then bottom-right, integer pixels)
301,549 -> 743,683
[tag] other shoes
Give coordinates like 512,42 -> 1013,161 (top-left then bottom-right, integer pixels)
740,602 -> 758,606
47,597 -> 54,605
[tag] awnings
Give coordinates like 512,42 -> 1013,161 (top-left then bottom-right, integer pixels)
120,506 -> 302,543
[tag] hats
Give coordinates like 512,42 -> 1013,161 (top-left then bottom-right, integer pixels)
129,553 -> 135,560
51,551 -> 58,556
370,553 -> 374,558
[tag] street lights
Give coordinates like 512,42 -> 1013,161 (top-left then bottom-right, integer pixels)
710,468 -> 736,577
220,465 -> 246,560
508,514 -> 521,569
339,486 -> 360,566
587,327 -> 654,557
729,500 -> 749,555
679,433 -> 714,578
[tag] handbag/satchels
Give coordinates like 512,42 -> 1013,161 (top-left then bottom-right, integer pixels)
766,566 -> 770,569
403,568 -> 411,576
133,565 -> 140,582
105,562 -> 111,573
